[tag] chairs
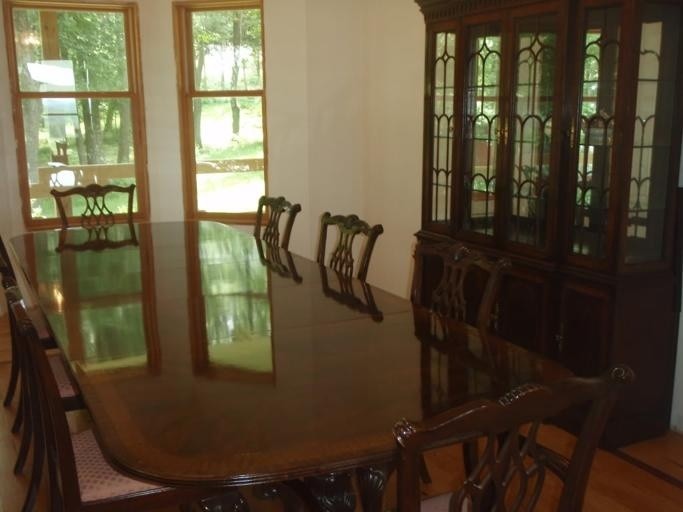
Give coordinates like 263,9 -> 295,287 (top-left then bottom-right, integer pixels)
50,182 -> 137,228
0,235 -> 246,510
316,212 -> 385,282
253,195 -> 302,249
410,230 -> 512,331
390,360 -> 631,511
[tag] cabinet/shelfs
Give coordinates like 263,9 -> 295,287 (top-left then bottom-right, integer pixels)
407,1 -> 682,448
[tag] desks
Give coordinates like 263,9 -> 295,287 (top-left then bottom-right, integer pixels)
10,222 -> 593,510
